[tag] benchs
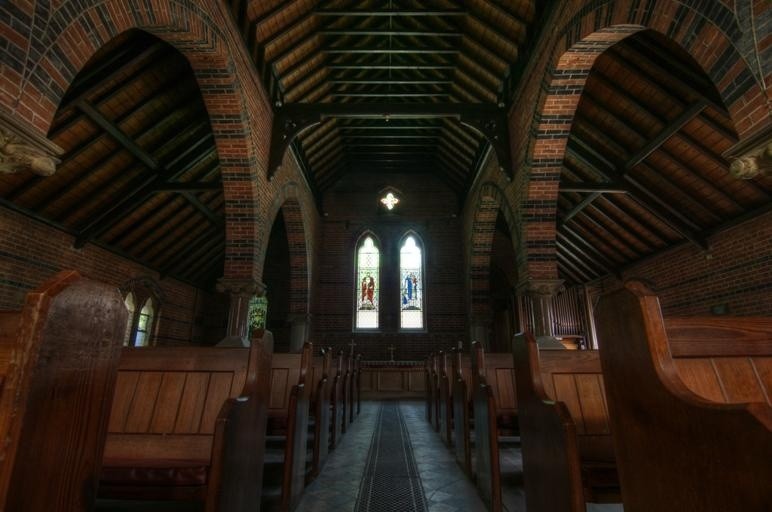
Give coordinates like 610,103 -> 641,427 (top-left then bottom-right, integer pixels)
594,281 -> 768,510
96,329 -> 360,512
425,341 -> 515,511
2,271 -> 129,511
513,333 -> 621,511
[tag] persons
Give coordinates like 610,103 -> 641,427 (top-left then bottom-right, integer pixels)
361,272 -> 375,305
404,272 -> 417,306
